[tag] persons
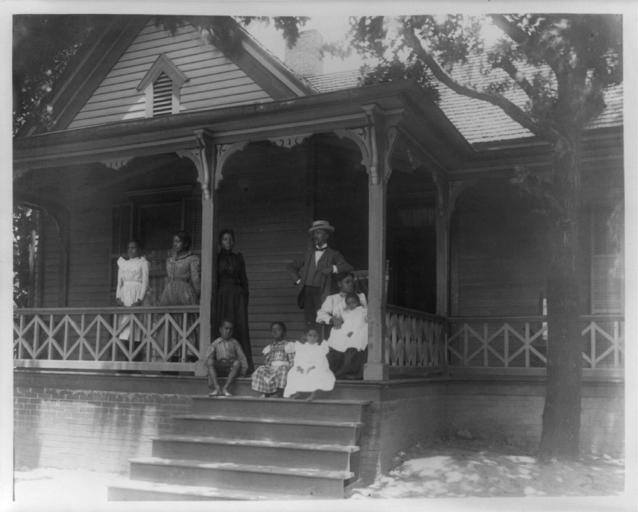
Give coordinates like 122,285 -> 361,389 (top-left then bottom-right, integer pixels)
117,233 -> 151,370
153,229 -> 201,375
289,221 -> 356,345
325,292 -> 367,353
250,321 -> 296,399
316,271 -> 367,379
216,229 -> 255,374
282,327 -> 336,400
208,319 -> 249,396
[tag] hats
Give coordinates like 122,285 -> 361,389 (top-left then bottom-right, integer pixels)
308,220 -> 335,232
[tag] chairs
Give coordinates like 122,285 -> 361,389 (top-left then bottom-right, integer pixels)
319,269 -> 369,380
